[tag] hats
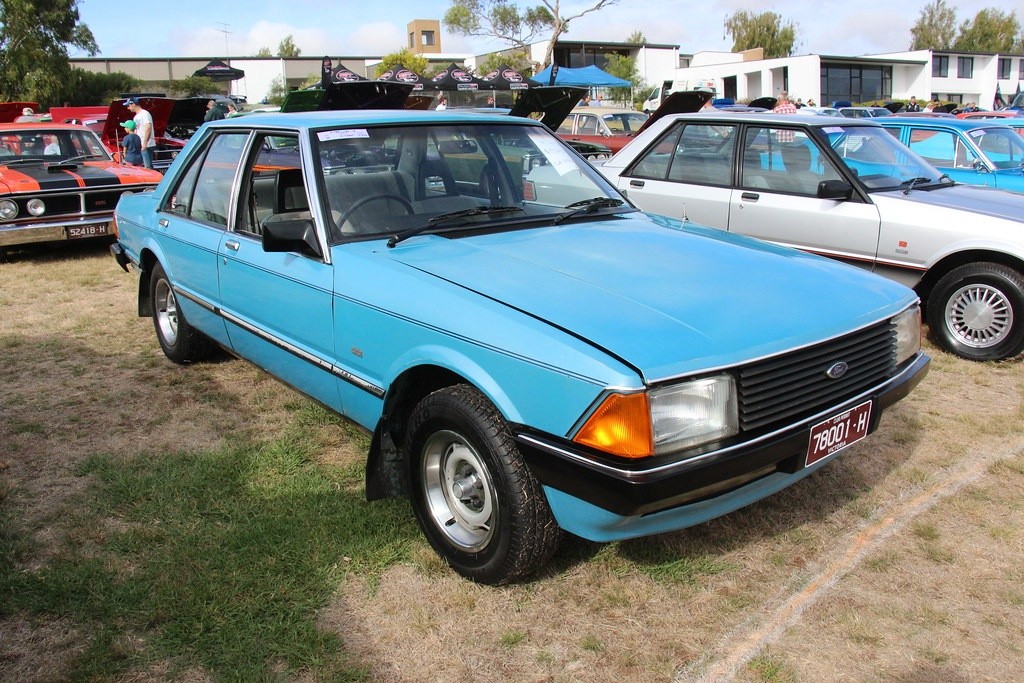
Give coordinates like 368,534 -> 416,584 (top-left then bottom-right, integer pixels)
120,119 -> 137,130
122,97 -> 138,105
22,107 -> 34,116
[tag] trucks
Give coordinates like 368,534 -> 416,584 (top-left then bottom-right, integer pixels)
643,77 -> 718,113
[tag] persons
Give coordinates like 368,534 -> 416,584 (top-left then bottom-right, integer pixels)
906,96 -> 919,112
436,98 -> 447,110
120,97 -> 156,170
228,103 -> 237,117
64,102 -> 70,107
576,94 -> 603,129
794,98 -> 806,109
487,96 -> 494,107
923,98 -> 947,118
14,108 -> 61,155
773,92 -> 797,148
204,101 -> 225,123
869,101 -> 881,117
964,102 -> 980,113
698,88 -> 729,146
808,99 -> 815,107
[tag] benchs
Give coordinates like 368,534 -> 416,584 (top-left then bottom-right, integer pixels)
642,152 -> 762,184
191,169 -> 417,234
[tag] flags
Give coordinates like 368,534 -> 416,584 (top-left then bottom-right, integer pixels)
1011,84 -> 1022,106
994,83 -> 1006,110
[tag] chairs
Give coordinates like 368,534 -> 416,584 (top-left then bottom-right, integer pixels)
779,145 -> 823,194
744,148 -> 779,191
259,168 -> 356,233
403,160 -> 481,215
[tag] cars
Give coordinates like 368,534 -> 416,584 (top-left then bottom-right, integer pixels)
800,107 -> 839,117
120,93 -> 216,139
381,129 -> 615,202
756,116 -> 1024,197
711,98 -> 735,109
227,94 -> 247,105
225,89 -> 327,149
262,99 -> 270,104
880,112 -> 955,144
111,107 -> 930,585
906,119 -> 1024,167
63,98 -> 187,175
527,107 -> 685,159
199,94 -> 239,119
0,123 -> 164,264
762,109 -> 846,138
185,111 -> 349,186
957,110 -> 1020,119
831,100 -> 851,109
839,107 -> 893,119
521,113 -> 1024,362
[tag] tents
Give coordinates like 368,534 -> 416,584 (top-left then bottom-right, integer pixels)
531,64 -> 633,115
303,60 -> 543,107
190,57 -> 247,102
